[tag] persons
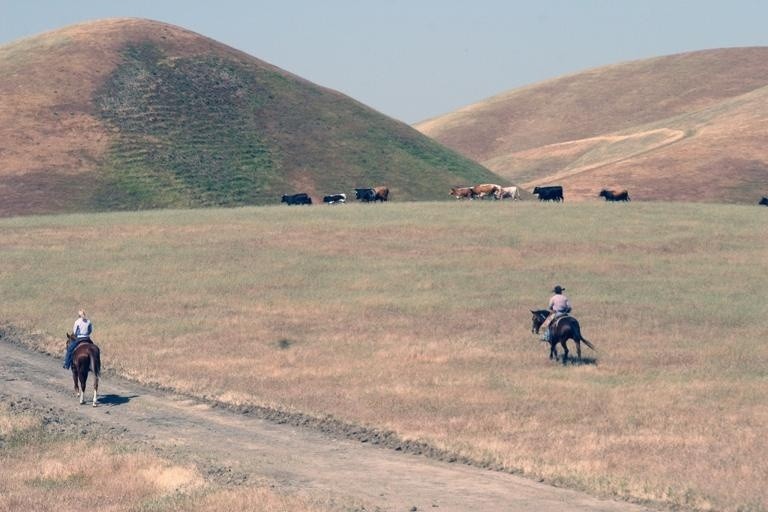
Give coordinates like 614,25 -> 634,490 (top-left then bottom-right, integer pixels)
536,285 -> 567,342
62,308 -> 92,370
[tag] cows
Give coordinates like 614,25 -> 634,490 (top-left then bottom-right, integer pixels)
758,196 -> 768,207
281,193 -> 312,205
533,186 -> 564,204
600,188 -> 631,202
354,185 -> 389,202
323,193 -> 346,204
448,183 -> 522,201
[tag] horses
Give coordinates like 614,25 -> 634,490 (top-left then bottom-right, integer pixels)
529,309 -> 598,366
66,332 -> 100,407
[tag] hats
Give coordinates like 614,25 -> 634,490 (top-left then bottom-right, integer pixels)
78,308 -> 88,318
551,285 -> 566,293
